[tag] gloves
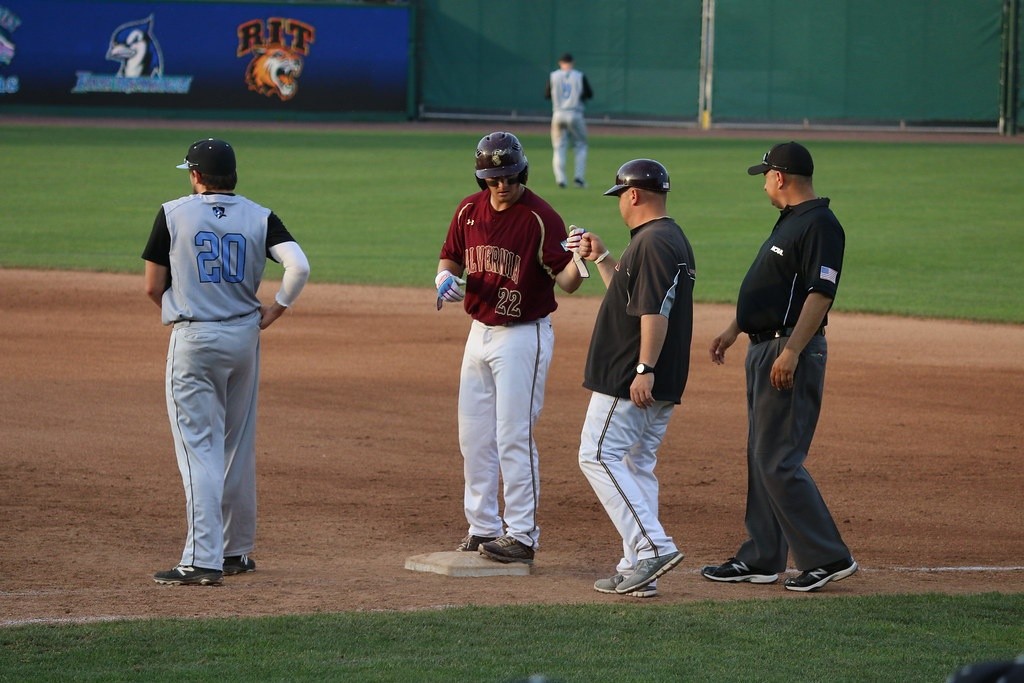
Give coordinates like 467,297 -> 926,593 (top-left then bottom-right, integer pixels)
435,269 -> 467,311
565,224 -> 590,278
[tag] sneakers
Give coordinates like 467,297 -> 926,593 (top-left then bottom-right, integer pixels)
701,557 -> 778,583
153,566 -> 223,586
594,573 -> 657,597
222,554 -> 255,575
615,551 -> 685,594
456,534 -> 502,552
784,555 -> 858,591
478,534 -> 535,565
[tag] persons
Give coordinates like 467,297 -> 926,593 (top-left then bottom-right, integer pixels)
577,159 -> 696,596
702,141 -> 859,590
140,138 -> 311,585
434,131 -> 586,564
544,53 -> 594,188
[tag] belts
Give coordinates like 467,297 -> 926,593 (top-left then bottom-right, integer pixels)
174,308 -> 258,324
749,327 -> 825,344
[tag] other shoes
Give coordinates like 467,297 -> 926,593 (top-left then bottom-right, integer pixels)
573,177 -> 588,188
558,182 -> 567,187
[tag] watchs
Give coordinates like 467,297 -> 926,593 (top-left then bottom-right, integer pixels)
635,363 -> 654,375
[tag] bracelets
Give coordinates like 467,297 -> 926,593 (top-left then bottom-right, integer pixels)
594,250 -> 609,265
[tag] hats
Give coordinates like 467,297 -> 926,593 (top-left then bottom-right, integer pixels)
748,141 -> 814,176
176,137 -> 236,176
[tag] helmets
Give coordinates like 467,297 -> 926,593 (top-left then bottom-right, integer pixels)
603,159 -> 671,196
474,132 -> 528,191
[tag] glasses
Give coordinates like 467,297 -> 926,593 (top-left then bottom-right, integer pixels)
184,155 -> 199,166
762,150 -> 788,170
